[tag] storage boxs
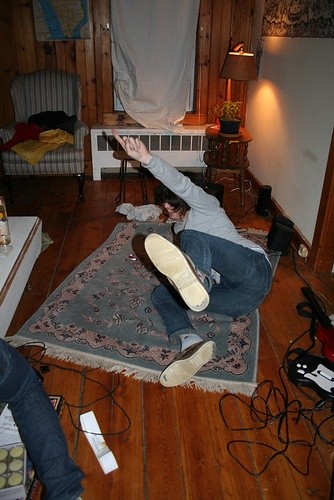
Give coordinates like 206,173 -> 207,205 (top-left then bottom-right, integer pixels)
0,441 -> 28,500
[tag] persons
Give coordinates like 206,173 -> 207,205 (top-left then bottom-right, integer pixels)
111,128 -> 273,387
0,338 -> 86,500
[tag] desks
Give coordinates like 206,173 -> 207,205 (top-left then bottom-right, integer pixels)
0,216 -> 42,340
203,126 -> 253,206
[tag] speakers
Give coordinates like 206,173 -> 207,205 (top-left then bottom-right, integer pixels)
266,223 -> 293,257
271,213 -> 294,229
256,184 -> 272,214
202,182 -> 224,208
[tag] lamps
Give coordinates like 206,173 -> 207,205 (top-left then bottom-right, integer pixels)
219,42 -> 258,102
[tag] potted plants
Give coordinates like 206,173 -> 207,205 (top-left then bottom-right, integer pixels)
210,101 -> 243,134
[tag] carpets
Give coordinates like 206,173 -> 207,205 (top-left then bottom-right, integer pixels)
2,224 -> 281,398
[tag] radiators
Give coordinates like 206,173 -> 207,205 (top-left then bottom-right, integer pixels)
91,126 -> 214,181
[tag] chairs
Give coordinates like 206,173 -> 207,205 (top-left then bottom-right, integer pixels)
0,68 -> 89,200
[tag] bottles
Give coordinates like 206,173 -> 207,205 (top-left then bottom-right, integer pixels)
0,196 -> 11,246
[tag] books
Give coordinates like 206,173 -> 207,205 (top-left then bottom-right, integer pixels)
0,394 -> 64,500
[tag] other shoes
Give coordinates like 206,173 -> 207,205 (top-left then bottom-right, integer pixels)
143,233 -> 210,312
159,339 -> 217,388
